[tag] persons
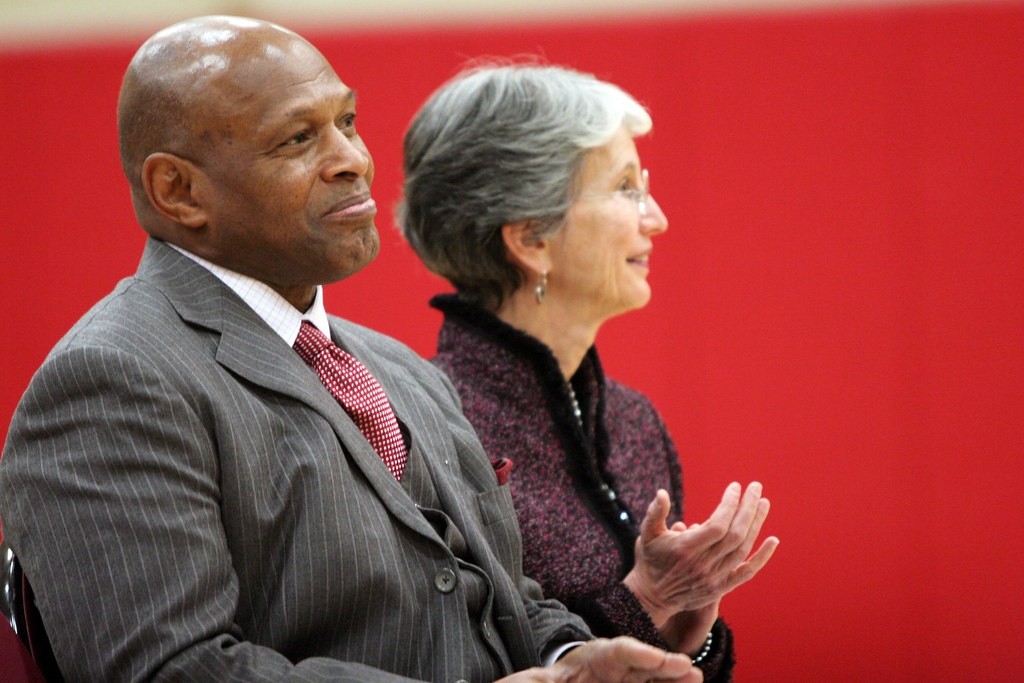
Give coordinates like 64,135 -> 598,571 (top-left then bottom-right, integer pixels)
394,64 -> 779,683
1,14 -> 705,682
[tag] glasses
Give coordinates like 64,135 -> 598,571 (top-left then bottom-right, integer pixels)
565,168 -> 651,217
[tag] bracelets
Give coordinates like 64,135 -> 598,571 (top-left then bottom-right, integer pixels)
689,632 -> 712,665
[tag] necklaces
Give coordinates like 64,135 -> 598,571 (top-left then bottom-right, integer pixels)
564,383 -> 627,520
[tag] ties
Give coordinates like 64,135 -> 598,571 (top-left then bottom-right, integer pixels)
292,320 -> 409,483
